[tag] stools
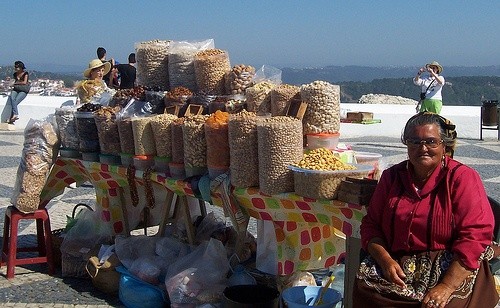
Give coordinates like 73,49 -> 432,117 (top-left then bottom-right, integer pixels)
0,206 -> 55,280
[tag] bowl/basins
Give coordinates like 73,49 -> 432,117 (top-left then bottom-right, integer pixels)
59,149 -> 232,181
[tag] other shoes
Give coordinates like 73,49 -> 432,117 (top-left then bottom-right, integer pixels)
10,116 -> 18,121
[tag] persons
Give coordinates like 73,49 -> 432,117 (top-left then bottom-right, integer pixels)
84,59 -> 111,88
352,111 -> 500,308
7,61 -> 31,124
413,60 -> 445,113
116,53 -> 136,90
96,47 -> 115,87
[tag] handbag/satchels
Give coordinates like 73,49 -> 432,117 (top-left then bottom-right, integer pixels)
165,238 -> 229,303
14,83 -> 30,93
420,92 -> 426,100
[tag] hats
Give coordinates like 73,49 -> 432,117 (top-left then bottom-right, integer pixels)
83,59 -> 111,77
426,61 -> 443,74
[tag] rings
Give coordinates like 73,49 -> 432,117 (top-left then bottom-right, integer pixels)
429,299 -> 439,308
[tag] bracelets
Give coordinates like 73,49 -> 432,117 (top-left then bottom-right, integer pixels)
418,72 -> 420,75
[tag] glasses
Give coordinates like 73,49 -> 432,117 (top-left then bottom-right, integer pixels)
409,137 -> 442,148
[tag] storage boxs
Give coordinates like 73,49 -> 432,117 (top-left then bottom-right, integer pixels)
62,251 -> 89,277
286,162 -> 374,200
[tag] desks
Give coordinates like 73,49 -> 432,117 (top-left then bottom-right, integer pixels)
40,157 -> 386,308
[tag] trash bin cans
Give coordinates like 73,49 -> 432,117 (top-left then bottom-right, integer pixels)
481,100 -> 500,126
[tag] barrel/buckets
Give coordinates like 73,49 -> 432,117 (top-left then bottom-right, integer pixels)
281,285 -> 343,308
223,283 -> 281,308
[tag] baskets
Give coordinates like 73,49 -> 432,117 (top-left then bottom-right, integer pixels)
51,204 -> 93,265
